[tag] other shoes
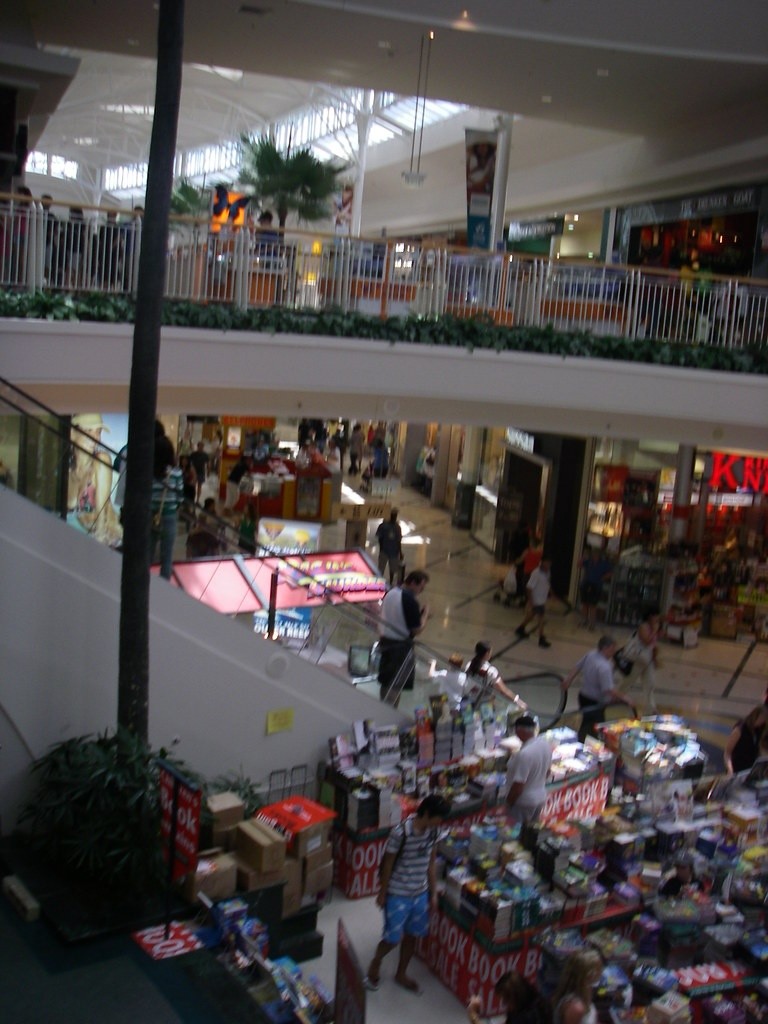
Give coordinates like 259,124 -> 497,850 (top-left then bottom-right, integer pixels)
393,973 -> 419,991
579,620 -> 596,632
515,625 -> 529,639
366,964 -> 380,991
538,637 -> 551,648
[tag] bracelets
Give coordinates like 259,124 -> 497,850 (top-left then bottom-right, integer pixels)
513,695 -> 519,704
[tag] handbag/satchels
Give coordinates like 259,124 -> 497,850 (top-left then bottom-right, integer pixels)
504,564 -> 517,594
610,647 -> 633,674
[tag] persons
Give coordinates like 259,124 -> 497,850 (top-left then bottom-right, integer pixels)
576,545 -> 612,632
428,639 -> 528,712
515,552 -> 553,648
0,186 -> 144,293
367,794 -> 450,991
606,606 -> 664,716
656,524 -> 733,648
0,458 -> 15,490
516,825 -> 705,900
559,635 -> 641,740
67,412 -> 127,550
723,686 -> 768,780
255,211 -> 281,270
298,417 -> 399,479
375,508 -> 405,584
468,942 -> 633,1024
150,418 -> 289,582
516,535 -> 544,607
416,441 -> 436,497
503,716 -> 552,827
668,791 -> 679,826
377,569 -> 432,709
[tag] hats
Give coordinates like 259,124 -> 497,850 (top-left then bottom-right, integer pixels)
511,717 -> 535,728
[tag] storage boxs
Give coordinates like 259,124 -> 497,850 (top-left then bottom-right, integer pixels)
184,791 -> 334,921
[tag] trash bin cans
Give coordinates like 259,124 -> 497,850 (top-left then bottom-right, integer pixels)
186,532 -> 216,555
452,485 -> 475,529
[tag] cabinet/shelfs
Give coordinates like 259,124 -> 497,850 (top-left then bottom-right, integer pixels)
575,559 -> 701,646
